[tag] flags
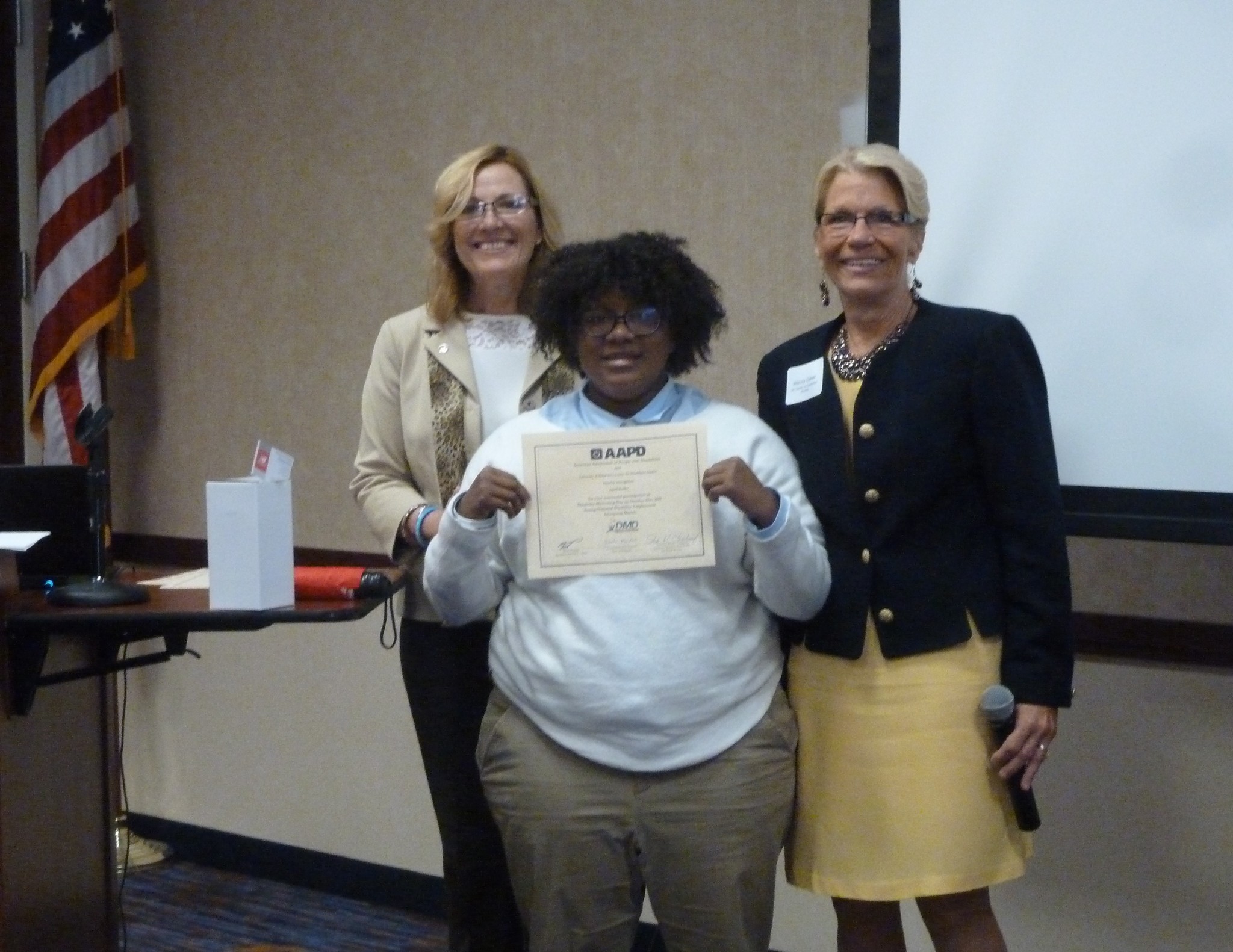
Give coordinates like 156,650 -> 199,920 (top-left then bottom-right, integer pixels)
25,0 -> 149,547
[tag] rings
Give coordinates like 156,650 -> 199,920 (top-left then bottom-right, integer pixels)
1037,743 -> 1048,754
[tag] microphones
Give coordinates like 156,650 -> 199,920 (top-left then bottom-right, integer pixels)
975,681 -> 1047,833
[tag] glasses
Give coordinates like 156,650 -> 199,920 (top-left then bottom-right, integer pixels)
449,194 -> 536,220
817,210 -> 905,237
575,306 -> 667,339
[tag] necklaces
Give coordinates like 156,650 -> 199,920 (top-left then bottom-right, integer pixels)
831,298 -> 916,382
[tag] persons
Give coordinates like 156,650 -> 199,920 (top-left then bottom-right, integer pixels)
759,141 -> 1073,952
423,231 -> 831,952
351,143 -> 582,952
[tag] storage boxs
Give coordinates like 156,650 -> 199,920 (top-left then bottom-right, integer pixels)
204,478 -> 295,611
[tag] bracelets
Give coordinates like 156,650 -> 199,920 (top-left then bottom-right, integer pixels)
416,507 -> 437,548
400,505 -> 419,545
